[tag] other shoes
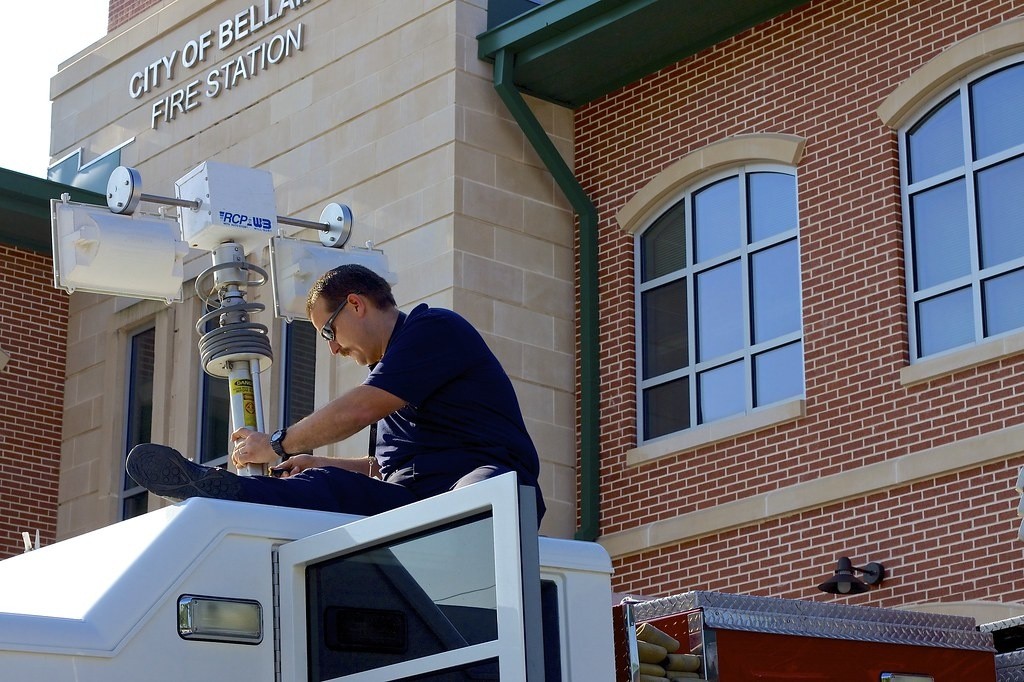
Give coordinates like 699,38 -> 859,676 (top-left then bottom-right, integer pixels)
126,443 -> 242,503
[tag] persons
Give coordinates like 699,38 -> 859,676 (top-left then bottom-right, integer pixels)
124,263 -> 548,532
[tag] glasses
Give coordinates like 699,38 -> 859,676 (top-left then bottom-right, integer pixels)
320,288 -> 360,341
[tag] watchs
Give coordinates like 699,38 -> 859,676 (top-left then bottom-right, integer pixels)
270,428 -> 293,459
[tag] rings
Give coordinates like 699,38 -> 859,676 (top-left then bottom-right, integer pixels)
237,448 -> 242,454
